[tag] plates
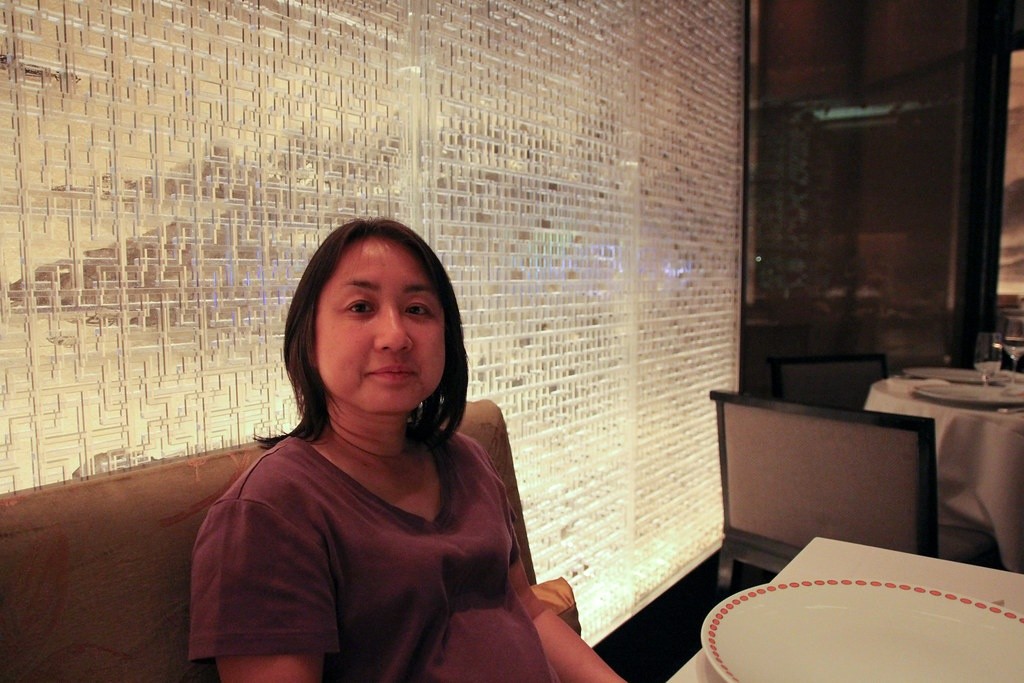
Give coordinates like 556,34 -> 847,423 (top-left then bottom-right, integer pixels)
700,575 -> 1024,683
902,367 -> 1010,383
912,382 -> 1024,406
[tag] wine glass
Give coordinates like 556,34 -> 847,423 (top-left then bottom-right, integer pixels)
1003,316 -> 1024,388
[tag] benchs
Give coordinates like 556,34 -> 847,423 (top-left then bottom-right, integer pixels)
0,400 -> 583,683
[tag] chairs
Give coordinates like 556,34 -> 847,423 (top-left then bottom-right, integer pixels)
709,390 -> 998,606
764,352 -> 889,411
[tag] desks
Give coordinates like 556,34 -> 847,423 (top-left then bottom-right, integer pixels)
861,367 -> 1024,573
665,536 -> 1024,683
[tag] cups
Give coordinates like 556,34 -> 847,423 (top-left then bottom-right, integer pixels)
974,332 -> 1003,378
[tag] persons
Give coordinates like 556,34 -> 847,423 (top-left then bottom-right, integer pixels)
186,219 -> 630,683
